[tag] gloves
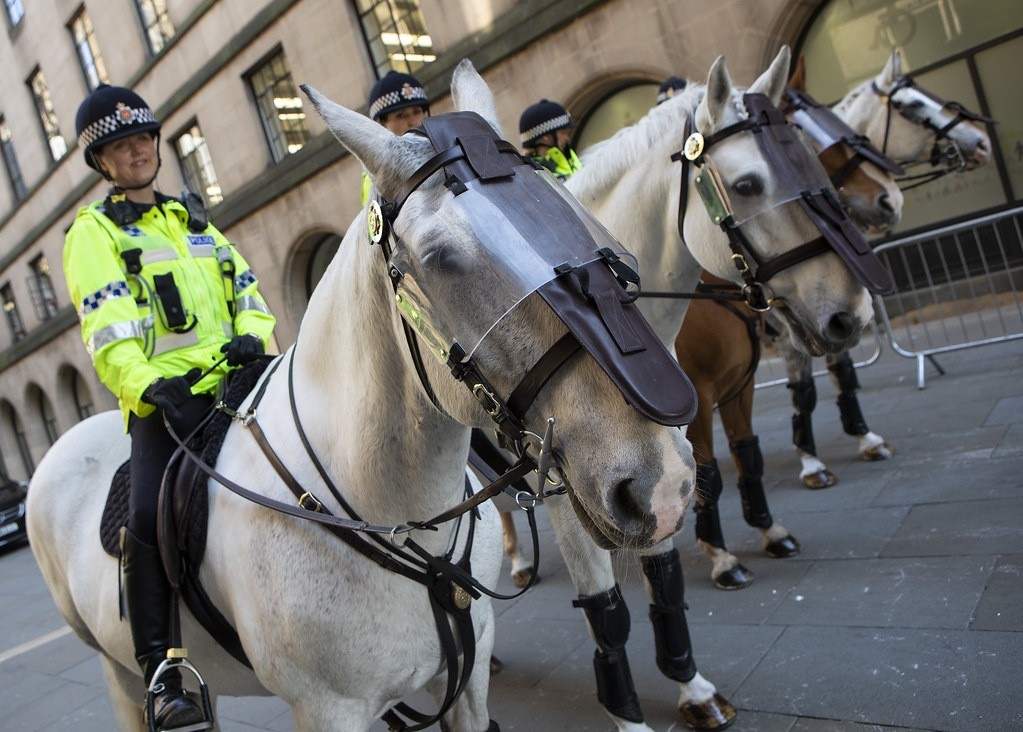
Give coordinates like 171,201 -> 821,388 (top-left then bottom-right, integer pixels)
220,336 -> 265,366
140,367 -> 203,419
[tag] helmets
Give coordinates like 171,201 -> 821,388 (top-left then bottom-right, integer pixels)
75,84 -> 161,171
368,70 -> 429,121
519,99 -> 571,147
657,76 -> 688,104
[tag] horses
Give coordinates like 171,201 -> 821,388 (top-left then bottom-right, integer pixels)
30,60 -> 695,729
465,48 -> 993,730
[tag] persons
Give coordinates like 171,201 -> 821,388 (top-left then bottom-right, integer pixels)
652,76 -> 688,105
361,71 -> 430,210
518,99 -> 582,185
62,83 -> 276,732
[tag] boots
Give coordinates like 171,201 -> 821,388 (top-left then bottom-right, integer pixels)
118,525 -> 213,732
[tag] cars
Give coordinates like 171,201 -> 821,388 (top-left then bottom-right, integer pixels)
0,473 -> 28,551
